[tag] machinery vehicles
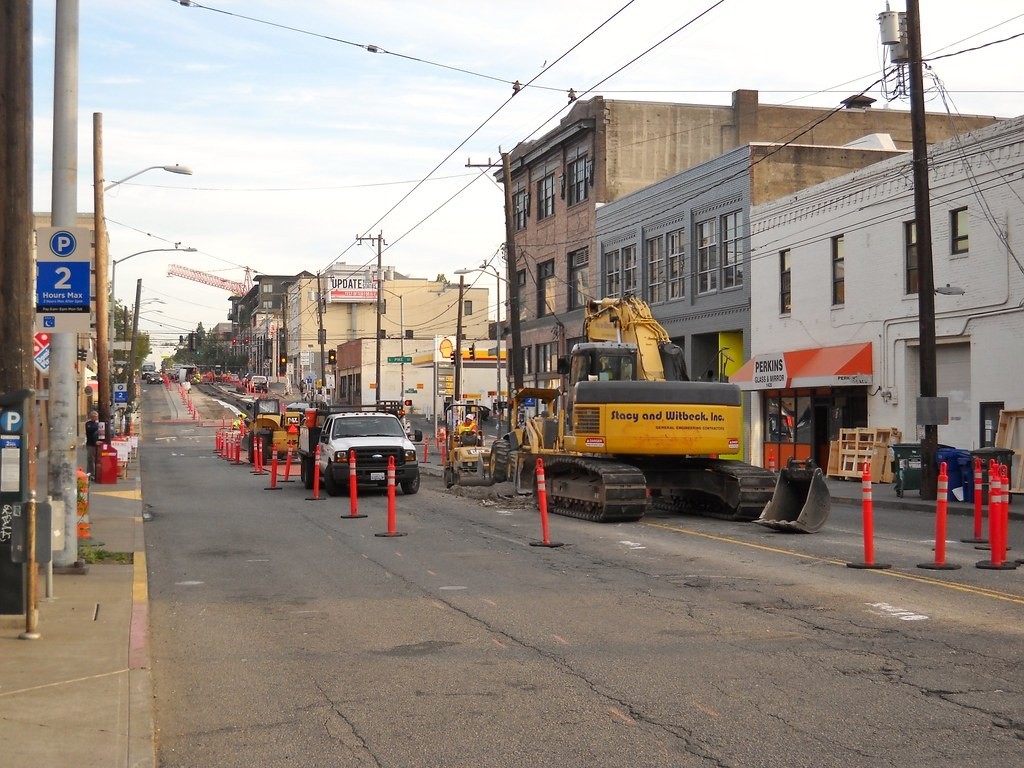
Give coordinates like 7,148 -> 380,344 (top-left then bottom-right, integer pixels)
444,403 -> 492,489
489,387 -> 557,496
246,398 -> 305,466
212,364 -> 223,382
532,295 -> 777,522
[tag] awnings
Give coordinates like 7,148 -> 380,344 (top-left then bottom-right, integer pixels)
729,343 -> 872,391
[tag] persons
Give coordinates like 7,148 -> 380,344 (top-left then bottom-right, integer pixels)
456,414 -> 477,469
227,371 -> 231,376
194,371 -> 200,382
300,380 -> 306,393
85,411 -> 104,481
233,414 -> 249,427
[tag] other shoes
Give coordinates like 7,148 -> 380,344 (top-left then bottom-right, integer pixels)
89,475 -> 95,481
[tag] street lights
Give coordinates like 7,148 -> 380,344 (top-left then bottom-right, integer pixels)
91,162 -> 196,445
454,267 -> 524,390
108,247 -> 199,439
479,263 -> 501,439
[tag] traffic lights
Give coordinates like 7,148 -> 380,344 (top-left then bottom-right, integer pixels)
328,349 -> 336,364
280,353 -> 288,374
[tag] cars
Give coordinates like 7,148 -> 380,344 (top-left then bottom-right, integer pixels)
147,372 -> 160,384
242,372 -> 259,387
230,374 -> 240,383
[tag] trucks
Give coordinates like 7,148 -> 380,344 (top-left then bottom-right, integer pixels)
161,355 -> 200,385
141,360 -> 156,381
297,400 -> 422,497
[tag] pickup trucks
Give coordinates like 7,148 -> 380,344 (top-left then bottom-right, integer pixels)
249,376 -> 269,393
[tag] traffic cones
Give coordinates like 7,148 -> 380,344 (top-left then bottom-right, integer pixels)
363,283 -> 406,404
